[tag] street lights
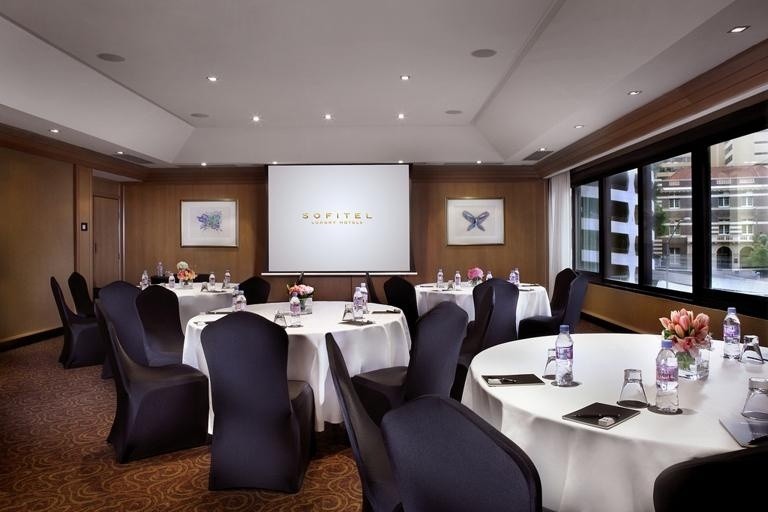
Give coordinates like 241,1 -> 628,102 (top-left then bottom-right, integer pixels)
666,216 -> 691,288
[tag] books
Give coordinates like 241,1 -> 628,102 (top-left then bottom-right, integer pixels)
519,288 -> 535,292
521,285 -> 540,287
207,310 -> 232,314
340,319 -> 375,325
207,290 -> 227,294
420,285 -> 433,287
195,320 -> 215,323
372,309 -> 400,313
482,373 -> 545,387
560,401 -> 640,430
432,288 -> 452,291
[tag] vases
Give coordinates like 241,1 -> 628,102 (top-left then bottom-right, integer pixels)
673,347 -> 709,382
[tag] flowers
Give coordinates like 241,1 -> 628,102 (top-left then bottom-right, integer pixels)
658,308 -> 712,371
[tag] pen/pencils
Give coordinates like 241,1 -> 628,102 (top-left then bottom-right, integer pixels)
573,413 -> 621,418
749,435 -> 768,443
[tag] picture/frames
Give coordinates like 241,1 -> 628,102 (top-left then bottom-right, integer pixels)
179,199 -> 240,248
444,196 -> 505,247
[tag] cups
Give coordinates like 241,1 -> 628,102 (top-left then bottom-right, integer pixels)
544,348 -> 555,380
341,303 -> 355,321
273,313 -> 287,327
618,369 -> 648,407
741,377 -> 768,422
739,335 -> 764,365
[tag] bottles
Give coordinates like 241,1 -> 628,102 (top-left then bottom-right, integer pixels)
168,272 -> 175,289
655,339 -> 680,414
209,272 -> 215,287
231,284 -> 247,312
142,270 -> 148,284
723,306 -> 740,358
352,282 -> 368,321
156,262 -> 163,276
555,324 -> 573,385
224,269 -> 230,283
436,268 -> 520,287
290,292 -> 300,326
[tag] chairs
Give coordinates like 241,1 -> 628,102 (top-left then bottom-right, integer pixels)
653,445 -> 768,512
